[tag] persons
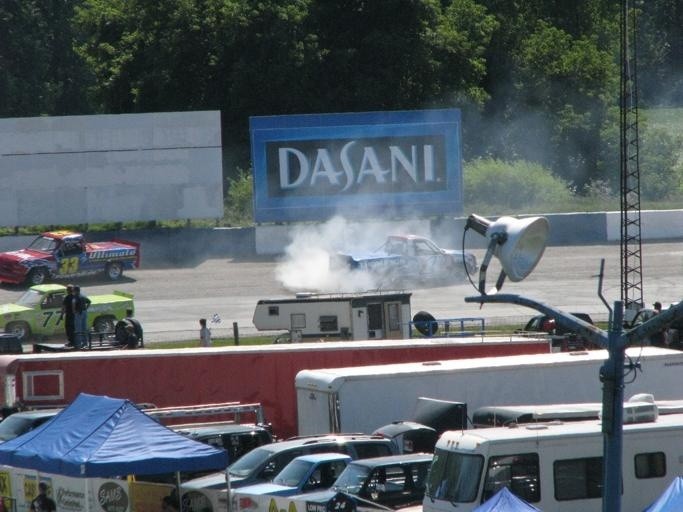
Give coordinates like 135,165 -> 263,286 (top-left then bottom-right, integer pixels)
652,301 -> 662,313
114,307 -> 142,345
119,323 -> 138,350
199,318 -> 211,346
161,488 -> 179,511
70,285 -> 90,350
647,309 -> 667,347
28,480 -> 56,511
61,284 -> 74,346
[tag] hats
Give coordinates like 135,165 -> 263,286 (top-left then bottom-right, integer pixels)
652,301 -> 661,307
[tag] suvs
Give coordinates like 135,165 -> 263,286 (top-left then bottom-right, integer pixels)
0,399 -> 467,511
514,312 -> 600,352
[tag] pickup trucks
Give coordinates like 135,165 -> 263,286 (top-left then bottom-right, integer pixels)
1,282 -> 136,339
326,234 -> 478,291
1,229 -> 140,289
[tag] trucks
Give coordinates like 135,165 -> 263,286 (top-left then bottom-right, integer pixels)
251,291 -> 414,344
0,333 -> 553,439
294,346 -> 683,453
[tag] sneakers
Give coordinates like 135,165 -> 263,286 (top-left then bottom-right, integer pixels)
65,342 -> 88,348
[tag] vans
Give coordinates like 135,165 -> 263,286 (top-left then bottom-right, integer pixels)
471,399 -> 683,479
422,392 -> 683,512
